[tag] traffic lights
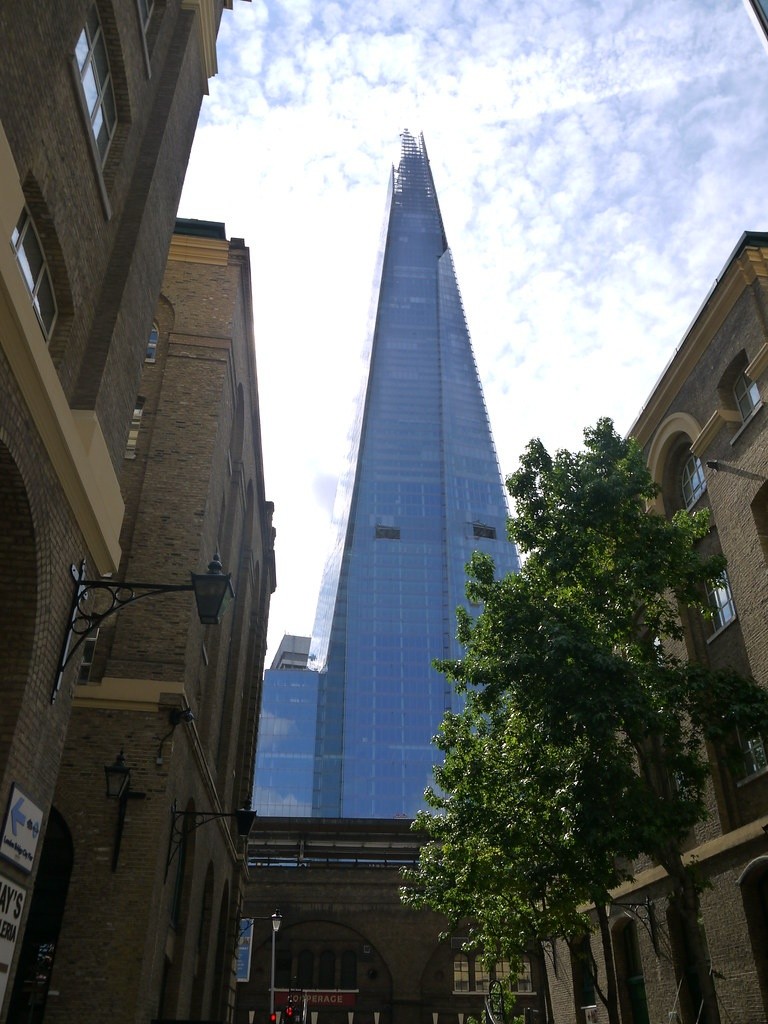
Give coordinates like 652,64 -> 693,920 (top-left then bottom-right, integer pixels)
269,1013 -> 277,1022
283,1006 -> 295,1024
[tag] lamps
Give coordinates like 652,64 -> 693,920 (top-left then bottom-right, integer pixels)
104,747 -> 146,871
49,554 -> 236,706
235,908 -> 282,951
605,899 -> 657,954
164,792 -> 259,886
